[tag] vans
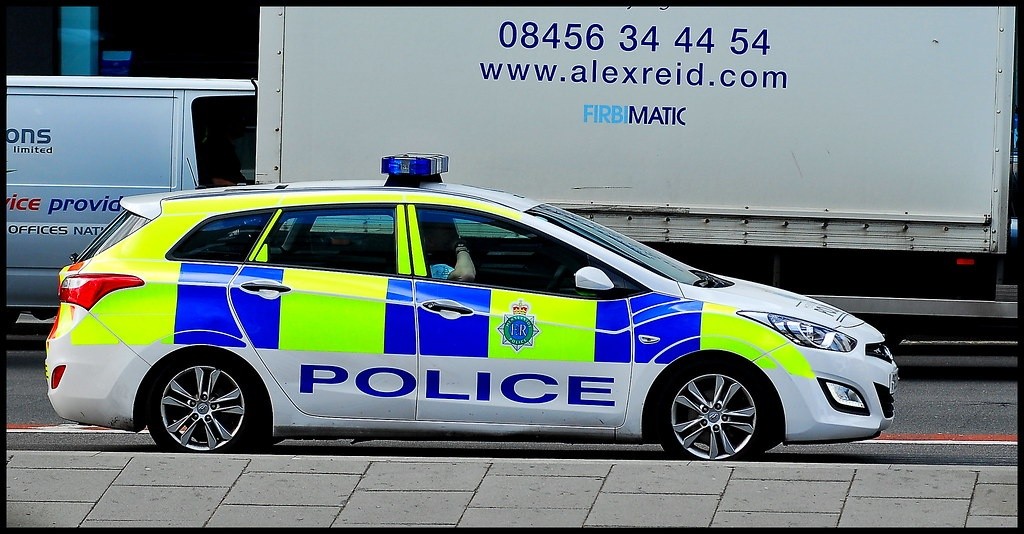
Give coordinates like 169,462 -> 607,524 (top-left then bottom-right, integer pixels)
6,71 -> 258,329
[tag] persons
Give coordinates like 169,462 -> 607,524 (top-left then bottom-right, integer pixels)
429,240 -> 475,283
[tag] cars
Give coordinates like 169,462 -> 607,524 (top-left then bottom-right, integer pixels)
44,153 -> 904,464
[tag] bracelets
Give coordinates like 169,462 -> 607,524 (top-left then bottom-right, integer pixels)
456,248 -> 470,255
455,241 -> 470,248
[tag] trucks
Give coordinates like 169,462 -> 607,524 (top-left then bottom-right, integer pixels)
250,4 -> 1023,344
